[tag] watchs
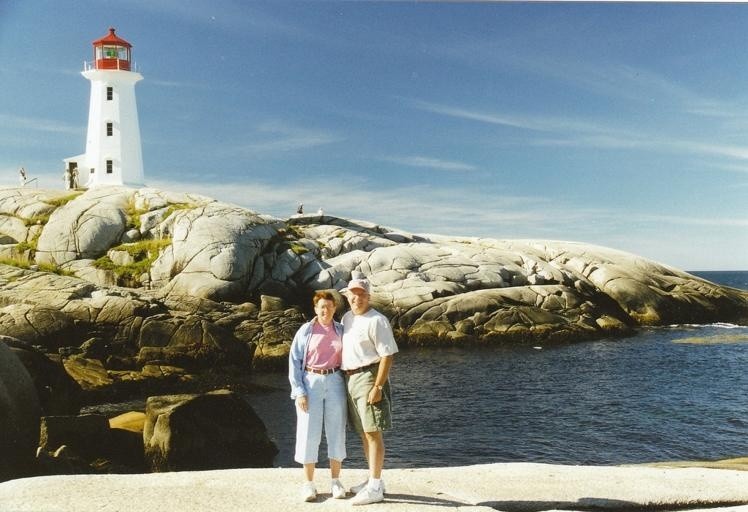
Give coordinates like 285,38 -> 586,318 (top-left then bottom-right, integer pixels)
375,384 -> 384,390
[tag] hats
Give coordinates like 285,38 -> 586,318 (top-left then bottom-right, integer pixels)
347,278 -> 370,291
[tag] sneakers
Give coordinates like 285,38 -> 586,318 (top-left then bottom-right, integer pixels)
303,483 -> 317,501
330,481 -> 346,499
349,478 -> 385,504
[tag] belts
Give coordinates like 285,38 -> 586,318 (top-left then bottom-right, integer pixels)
342,363 -> 379,376
305,366 -> 340,375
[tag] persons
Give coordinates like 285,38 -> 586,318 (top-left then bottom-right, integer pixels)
290,291 -> 348,501
338,278 -> 398,505
62,168 -> 72,192
297,203 -> 304,213
19,167 -> 27,186
319,207 -> 324,214
72,167 -> 80,190
